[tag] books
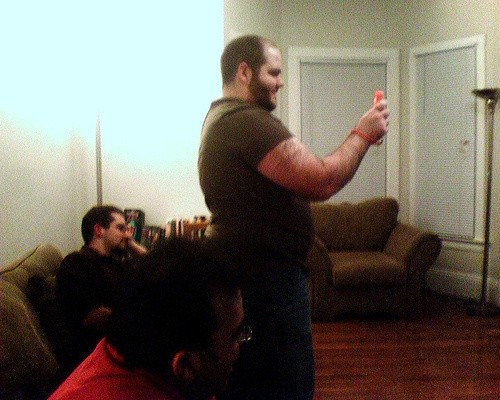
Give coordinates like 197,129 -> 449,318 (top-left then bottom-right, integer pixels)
125,209 -> 209,250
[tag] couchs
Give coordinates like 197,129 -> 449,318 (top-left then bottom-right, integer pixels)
0,244 -> 63,393
309,198 -> 442,323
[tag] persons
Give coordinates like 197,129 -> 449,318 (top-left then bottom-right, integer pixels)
48,237 -> 252,399
53,205 -> 147,368
199,35 -> 390,400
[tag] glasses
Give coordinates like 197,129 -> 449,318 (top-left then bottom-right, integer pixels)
238,317 -> 254,341
